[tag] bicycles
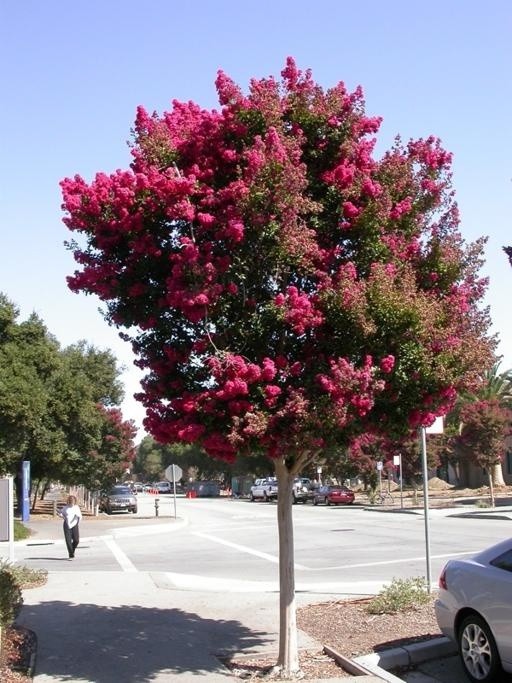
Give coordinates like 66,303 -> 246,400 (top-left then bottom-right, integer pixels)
372,484 -> 397,503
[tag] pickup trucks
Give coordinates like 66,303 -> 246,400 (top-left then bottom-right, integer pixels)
250,476 -> 355,505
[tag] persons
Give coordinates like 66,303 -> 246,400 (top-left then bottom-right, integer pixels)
56,495 -> 83,561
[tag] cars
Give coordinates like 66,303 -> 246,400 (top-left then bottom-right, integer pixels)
100,486 -> 137,513
435,535 -> 512,683
125,481 -> 172,492
